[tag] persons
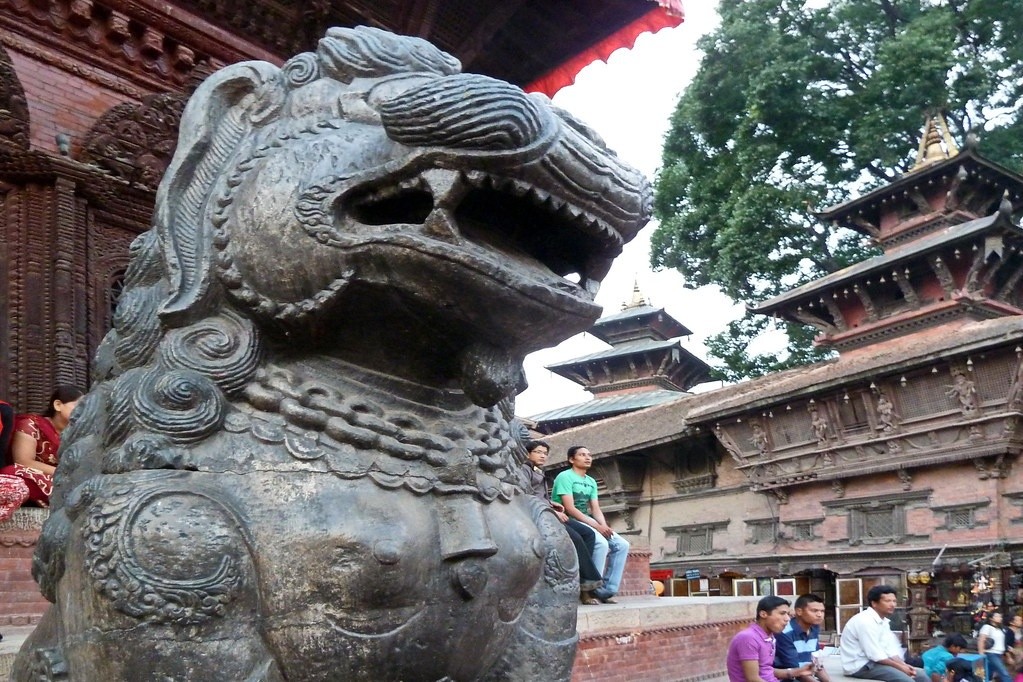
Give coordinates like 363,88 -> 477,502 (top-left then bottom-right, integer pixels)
550,445 -> 629,603
771,594 -> 830,682
841,585 -> 932,682
0,386 -> 86,521
524,441 -> 604,606
905,603 -> 1023,682
727,595 -> 816,682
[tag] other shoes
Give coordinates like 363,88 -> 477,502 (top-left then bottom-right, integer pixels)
580,579 -> 603,596
604,598 -> 618,603
580,592 -> 599,604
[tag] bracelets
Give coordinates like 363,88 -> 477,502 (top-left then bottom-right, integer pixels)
787,668 -> 795,679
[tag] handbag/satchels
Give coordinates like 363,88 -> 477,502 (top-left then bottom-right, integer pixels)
973,624 -> 994,651
